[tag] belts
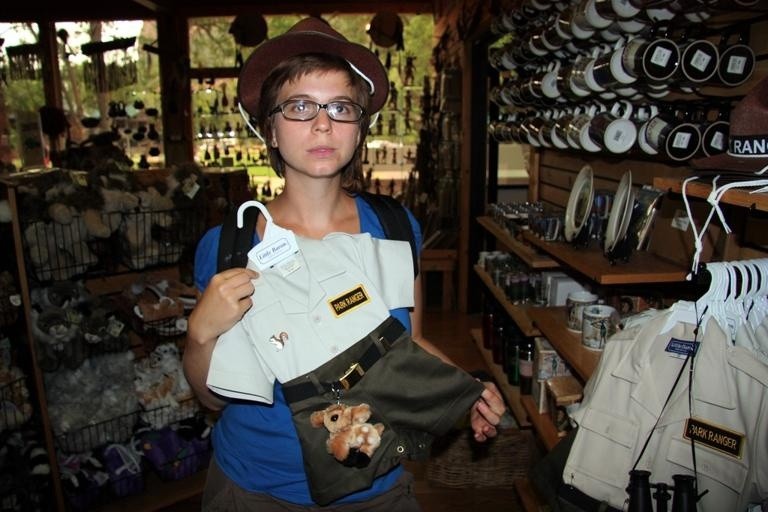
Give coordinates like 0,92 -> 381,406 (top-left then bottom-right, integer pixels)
280,316 -> 408,407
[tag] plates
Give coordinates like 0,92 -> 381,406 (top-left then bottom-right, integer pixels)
562,163 -> 596,244
601,169 -> 637,255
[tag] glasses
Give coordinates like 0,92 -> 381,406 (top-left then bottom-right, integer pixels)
268,98 -> 370,125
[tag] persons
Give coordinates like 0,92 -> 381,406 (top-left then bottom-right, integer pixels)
181,16 -> 507,512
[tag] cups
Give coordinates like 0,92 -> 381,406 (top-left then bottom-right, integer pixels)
475,0 -> 761,164
562,287 -> 619,350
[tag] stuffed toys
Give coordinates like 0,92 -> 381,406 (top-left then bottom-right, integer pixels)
310,401 -> 386,470
6,160 -> 255,494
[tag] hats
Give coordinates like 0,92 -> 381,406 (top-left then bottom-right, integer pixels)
688,78 -> 768,177
237,16 -> 388,118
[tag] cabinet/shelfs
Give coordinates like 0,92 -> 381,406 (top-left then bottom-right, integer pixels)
470,160 -> 768,509
0,169 -> 249,511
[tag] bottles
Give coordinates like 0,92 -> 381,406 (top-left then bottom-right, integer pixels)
474,297 -> 536,396
